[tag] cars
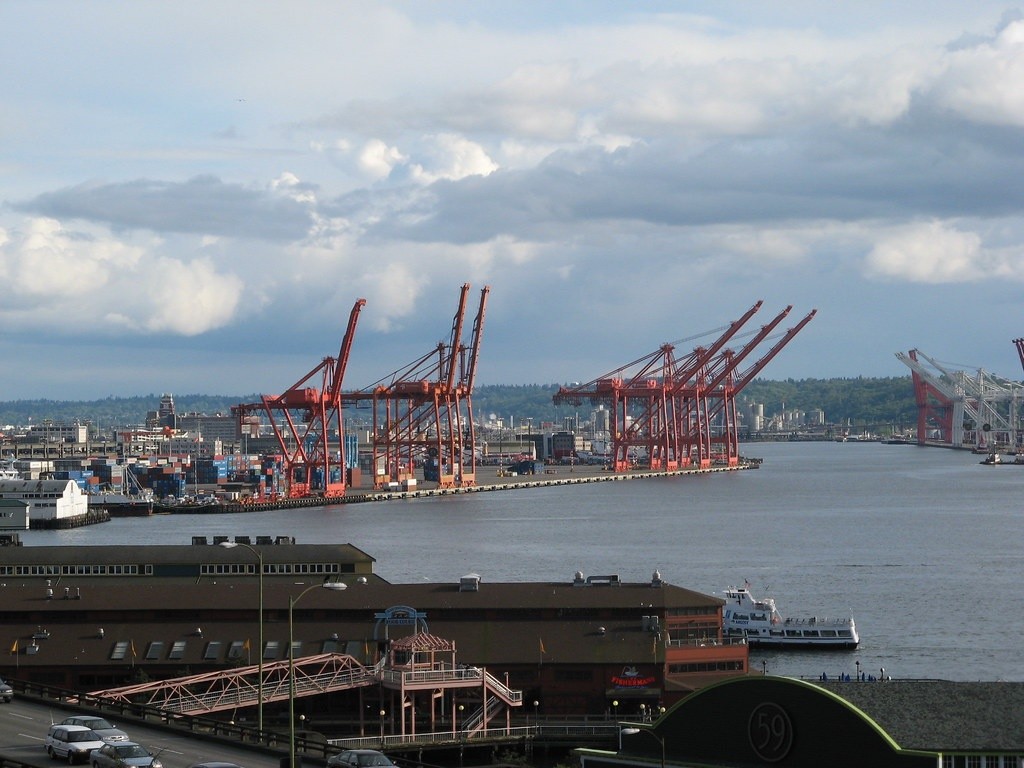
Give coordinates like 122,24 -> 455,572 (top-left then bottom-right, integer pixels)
44,715 -> 245,768
0,679 -> 14,704
326,749 -> 399,768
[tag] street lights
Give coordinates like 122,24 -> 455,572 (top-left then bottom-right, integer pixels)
621,727 -> 665,768
288,582 -> 348,768
568,417 -> 576,471
527,417 -> 534,462
218,541 -> 263,743
496,418 -> 504,476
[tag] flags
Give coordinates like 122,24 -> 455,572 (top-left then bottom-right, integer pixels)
540,639 -> 544,651
243,641 -> 251,649
8,640 -> 17,656
130,639 -> 136,657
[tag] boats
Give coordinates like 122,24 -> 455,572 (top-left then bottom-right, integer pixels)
979,441 -> 1024,465
834,427 -> 884,443
718,578 -> 860,648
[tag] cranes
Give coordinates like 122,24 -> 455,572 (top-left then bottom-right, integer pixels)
551,298 -> 819,471
230,282 -> 492,501
